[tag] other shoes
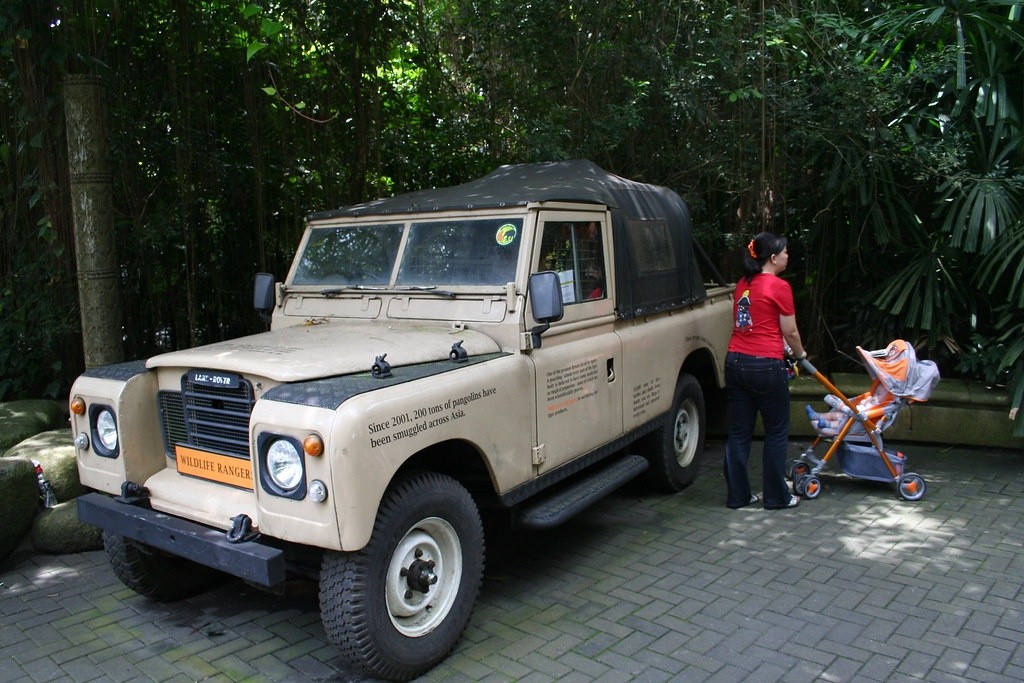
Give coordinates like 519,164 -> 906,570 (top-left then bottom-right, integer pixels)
746,493 -> 759,505
782,494 -> 800,508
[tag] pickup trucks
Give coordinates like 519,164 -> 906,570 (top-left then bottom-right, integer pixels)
67,160 -> 739,683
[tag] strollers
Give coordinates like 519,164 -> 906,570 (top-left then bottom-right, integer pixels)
787,340 -> 942,500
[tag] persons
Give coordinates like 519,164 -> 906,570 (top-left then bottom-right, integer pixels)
723,231 -> 807,510
805,383 -> 890,434
585,264 -> 604,301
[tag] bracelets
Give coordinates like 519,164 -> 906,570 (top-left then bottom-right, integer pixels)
787,348 -> 791,353
